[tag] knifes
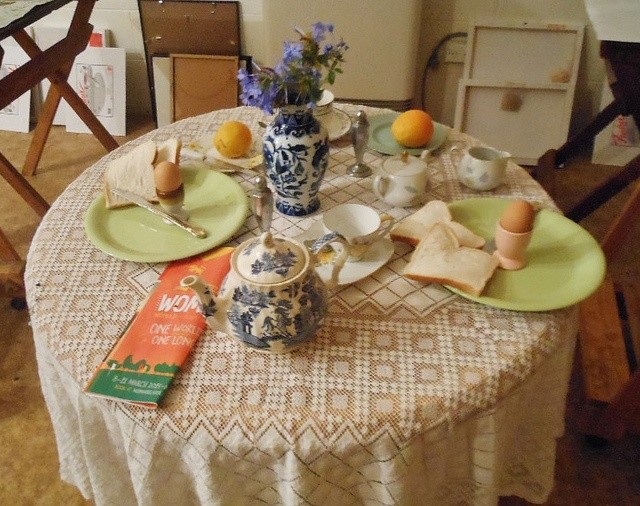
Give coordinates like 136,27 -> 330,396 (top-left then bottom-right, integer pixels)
177,148 -> 246,171
106,187 -> 209,240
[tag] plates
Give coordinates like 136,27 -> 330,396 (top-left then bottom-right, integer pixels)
416,196 -> 607,314
286,216 -> 395,287
316,103 -> 353,141
363,112 -> 447,158
83,161 -> 250,263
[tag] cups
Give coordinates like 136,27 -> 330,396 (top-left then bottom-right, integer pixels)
311,89 -> 335,127
491,219 -> 534,271
449,143 -> 513,192
322,202 -> 396,263
156,183 -> 189,225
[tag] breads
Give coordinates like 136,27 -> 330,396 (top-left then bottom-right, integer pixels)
103,142 -> 159,210
400,223 -> 501,297
390,199 -> 486,248
150,140 -> 184,169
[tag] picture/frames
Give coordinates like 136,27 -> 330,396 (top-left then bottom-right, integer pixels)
135,0 -> 254,128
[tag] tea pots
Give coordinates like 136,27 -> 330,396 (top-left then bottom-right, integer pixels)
179,229 -> 350,356
372,147 -> 432,208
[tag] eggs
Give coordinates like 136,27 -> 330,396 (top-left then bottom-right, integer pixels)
499,198 -> 535,232
154,163 -> 182,193
